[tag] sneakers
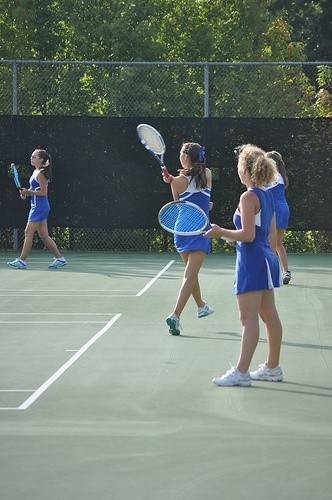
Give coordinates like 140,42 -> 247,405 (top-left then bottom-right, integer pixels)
282,270 -> 292,285
166,313 -> 184,336
7,258 -> 26,269
250,361 -> 284,381
48,257 -> 67,269
212,363 -> 253,387
198,302 -> 214,318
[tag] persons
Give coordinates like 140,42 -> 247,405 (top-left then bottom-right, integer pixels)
7,149 -> 68,270
261,152 -> 291,285
216,144 -> 283,384
162,143 -> 214,336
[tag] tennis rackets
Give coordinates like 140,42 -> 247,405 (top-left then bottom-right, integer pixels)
10,163 -> 23,192
136,123 -> 169,178
158,201 -> 234,242
